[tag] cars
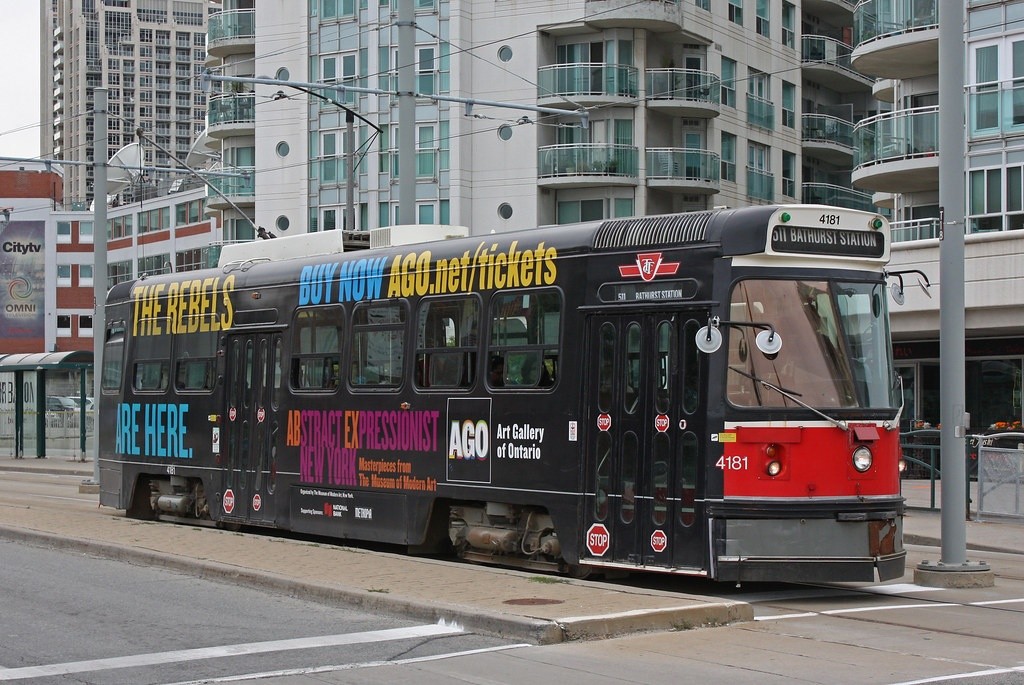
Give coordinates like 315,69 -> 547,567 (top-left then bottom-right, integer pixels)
45,395 -> 94,427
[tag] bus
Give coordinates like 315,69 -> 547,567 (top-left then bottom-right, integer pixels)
98,203 -> 932,584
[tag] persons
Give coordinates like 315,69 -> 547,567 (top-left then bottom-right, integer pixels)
417,354 -> 509,387
520,359 -> 554,387
322,357 -> 335,389
352,361 -> 366,385
794,305 -> 838,379
